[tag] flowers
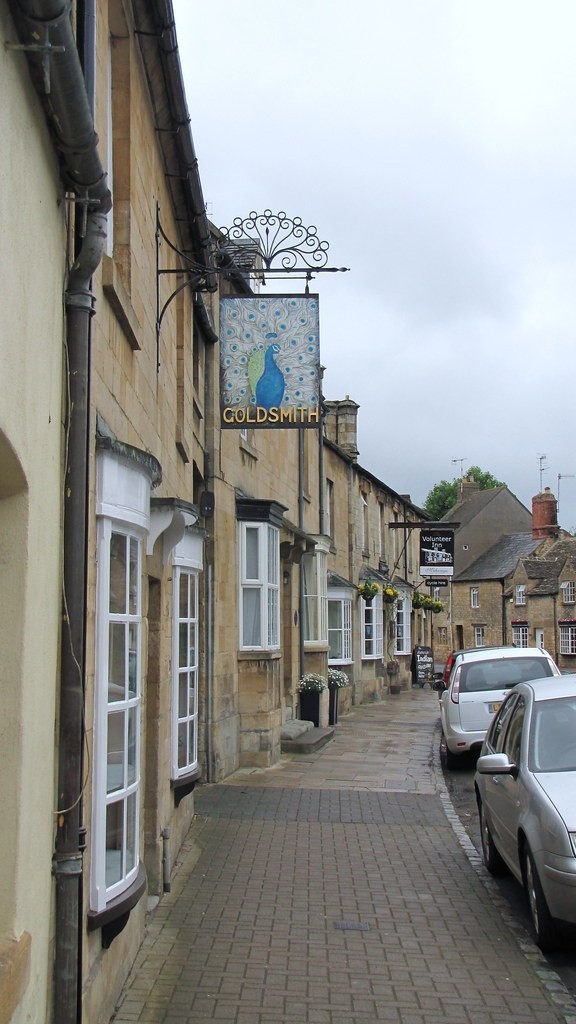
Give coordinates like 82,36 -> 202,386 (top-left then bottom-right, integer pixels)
411,593 -> 444,613
387,659 -> 398,666
295,672 -> 328,693
328,668 -> 351,689
383,586 -> 400,601
358,582 -> 380,597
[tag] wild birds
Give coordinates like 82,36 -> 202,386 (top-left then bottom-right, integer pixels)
221,298 -> 318,417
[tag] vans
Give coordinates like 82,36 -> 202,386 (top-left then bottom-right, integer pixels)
434,647 -> 561,771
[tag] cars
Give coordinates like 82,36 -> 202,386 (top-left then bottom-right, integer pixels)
473,671 -> 576,951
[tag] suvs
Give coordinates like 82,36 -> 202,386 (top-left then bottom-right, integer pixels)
432,644 -> 517,697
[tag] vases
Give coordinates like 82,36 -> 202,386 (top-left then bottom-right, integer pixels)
299,693 -> 322,728
390,685 -> 402,694
362,594 -> 374,601
384,598 -> 394,603
329,689 -> 338,725
413,602 -> 422,609
433,608 -> 441,613
388,665 -> 395,669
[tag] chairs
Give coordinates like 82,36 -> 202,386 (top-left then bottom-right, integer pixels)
538,705 -> 576,769
466,669 -> 491,689
